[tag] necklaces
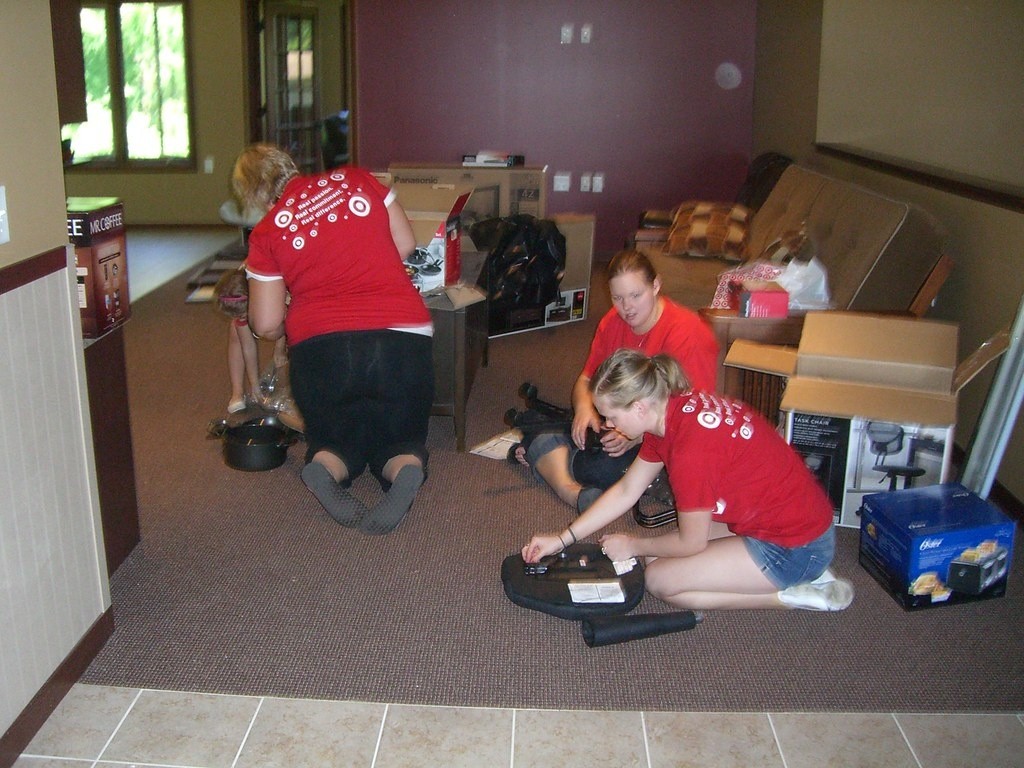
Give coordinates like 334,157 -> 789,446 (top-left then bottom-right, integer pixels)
637,307 -> 659,350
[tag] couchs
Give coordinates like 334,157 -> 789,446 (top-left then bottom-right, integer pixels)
622,152 -> 957,431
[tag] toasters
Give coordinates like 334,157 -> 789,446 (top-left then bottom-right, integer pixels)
944,546 -> 1009,593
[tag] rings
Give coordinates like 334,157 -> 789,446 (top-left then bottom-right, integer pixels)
601,548 -> 605,555
526,544 -> 530,546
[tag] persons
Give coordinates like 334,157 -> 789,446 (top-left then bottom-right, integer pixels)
515,250 -> 721,517
521,348 -> 854,613
214,255 -> 293,414
231,142 -> 435,536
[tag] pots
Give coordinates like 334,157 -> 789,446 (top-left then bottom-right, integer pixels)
208,418 -> 299,471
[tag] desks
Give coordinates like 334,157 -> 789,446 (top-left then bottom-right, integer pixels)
421,250 -> 490,452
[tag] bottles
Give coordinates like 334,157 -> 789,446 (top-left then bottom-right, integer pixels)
257,359 -> 308,435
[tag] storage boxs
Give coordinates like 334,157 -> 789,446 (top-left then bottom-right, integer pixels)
722,308 -> 1012,529
728,280 -> 789,319
859,479 -> 1017,612
366,163 -> 598,339
65,197 -> 132,339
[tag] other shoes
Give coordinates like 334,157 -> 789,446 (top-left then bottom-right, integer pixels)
227,394 -> 247,414
506,442 -> 533,469
576,487 -> 603,516
777,569 -> 854,612
359,464 -> 428,536
300,461 -> 369,528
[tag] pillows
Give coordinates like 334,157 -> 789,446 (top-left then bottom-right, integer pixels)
661,198 -> 752,261
757,221 -> 810,267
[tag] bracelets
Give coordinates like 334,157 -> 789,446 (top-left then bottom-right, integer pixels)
567,526 -> 577,544
558,535 -> 566,550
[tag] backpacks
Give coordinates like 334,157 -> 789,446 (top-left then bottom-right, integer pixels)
468,213 -> 567,331
